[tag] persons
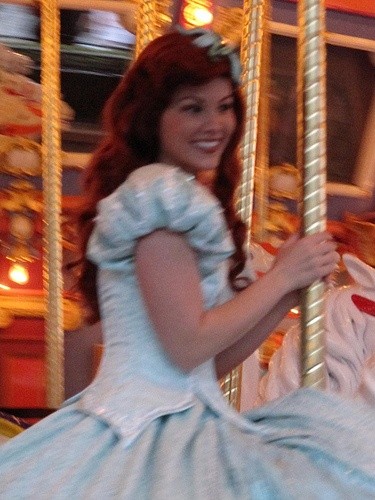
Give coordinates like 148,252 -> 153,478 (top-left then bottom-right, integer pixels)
0,24 -> 374,500
